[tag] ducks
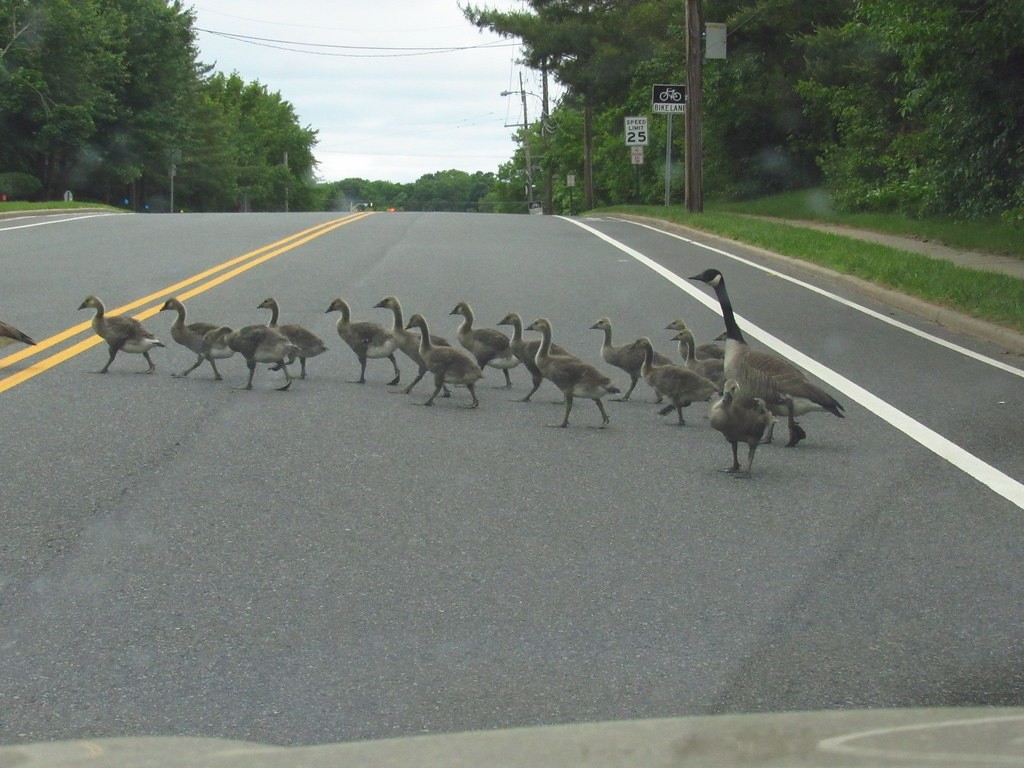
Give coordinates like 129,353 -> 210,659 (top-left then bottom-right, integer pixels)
0,269 -> 846,481
